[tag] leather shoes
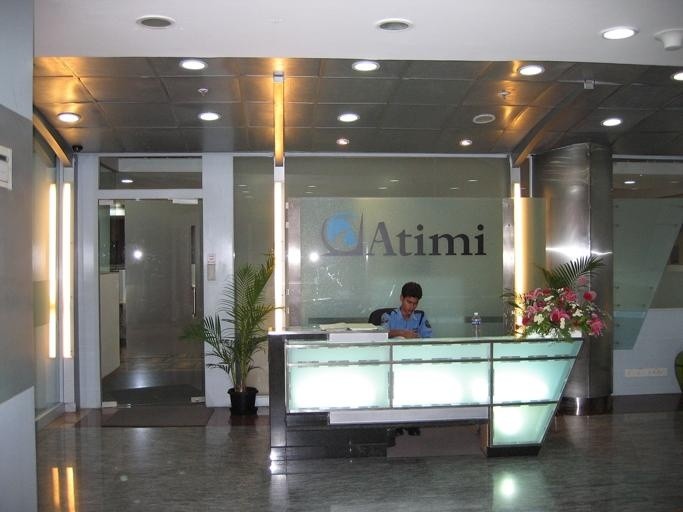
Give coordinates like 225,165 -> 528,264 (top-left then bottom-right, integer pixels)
405,426 -> 420,436
396,428 -> 404,436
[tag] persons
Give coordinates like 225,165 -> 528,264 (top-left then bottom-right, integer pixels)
379,281 -> 431,339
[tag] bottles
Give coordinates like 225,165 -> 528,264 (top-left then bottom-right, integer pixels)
471,312 -> 482,338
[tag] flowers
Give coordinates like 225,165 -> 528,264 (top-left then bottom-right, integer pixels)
499,253 -> 613,344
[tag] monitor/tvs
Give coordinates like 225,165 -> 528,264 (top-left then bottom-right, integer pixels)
326,331 -> 389,343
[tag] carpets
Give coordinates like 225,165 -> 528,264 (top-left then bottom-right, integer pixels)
108,383 -> 204,405
103,407 -> 215,427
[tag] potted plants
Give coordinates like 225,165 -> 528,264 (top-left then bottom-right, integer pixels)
175,247 -> 288,415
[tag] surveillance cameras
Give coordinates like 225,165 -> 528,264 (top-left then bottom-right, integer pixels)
72,146 -> 82,153
